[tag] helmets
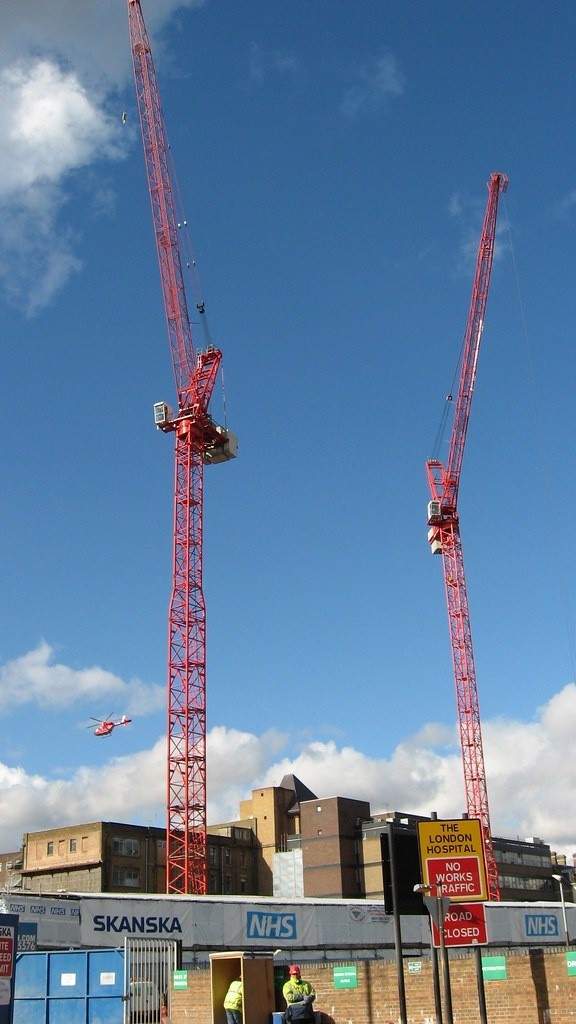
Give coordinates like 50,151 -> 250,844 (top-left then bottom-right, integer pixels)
289,966 -> 301,975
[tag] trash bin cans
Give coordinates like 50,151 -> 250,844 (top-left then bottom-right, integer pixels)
313,1011 -> 321,1024
272,1011 -> 286,1024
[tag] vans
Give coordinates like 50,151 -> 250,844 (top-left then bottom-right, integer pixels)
129,982 -> 159,1018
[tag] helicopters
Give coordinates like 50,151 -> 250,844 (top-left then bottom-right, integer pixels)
86,711 -> 132,738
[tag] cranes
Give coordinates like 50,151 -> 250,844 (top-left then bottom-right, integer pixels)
426,172 -> 509,902
121,1 -> 240,894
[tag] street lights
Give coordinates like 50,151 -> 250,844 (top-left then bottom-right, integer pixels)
551,874 -> 569,947
413,879 -> 453,1024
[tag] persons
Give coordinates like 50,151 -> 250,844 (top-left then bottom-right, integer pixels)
283,965 -> 317,1024
223,973 -> 244,1024
